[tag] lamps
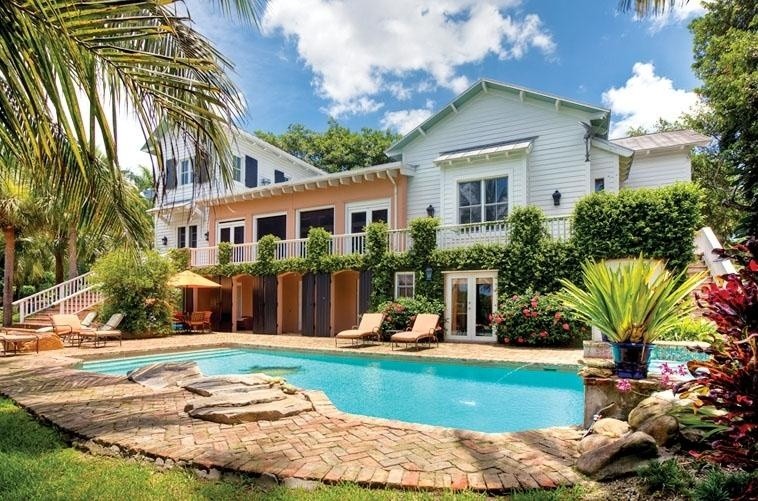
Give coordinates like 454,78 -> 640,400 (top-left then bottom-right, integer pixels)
552,189 -> 561,205
426,204 -> 434,218
161,236 -> 167,245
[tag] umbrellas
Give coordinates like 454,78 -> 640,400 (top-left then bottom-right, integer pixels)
164,271 -> 222,322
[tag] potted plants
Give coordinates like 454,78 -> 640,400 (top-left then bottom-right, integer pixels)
551,249 -> 713,379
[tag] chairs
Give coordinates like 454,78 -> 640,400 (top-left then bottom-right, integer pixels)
0,310 -> 124,358
176,310 -> 213,335
334,311 -> 441,353
